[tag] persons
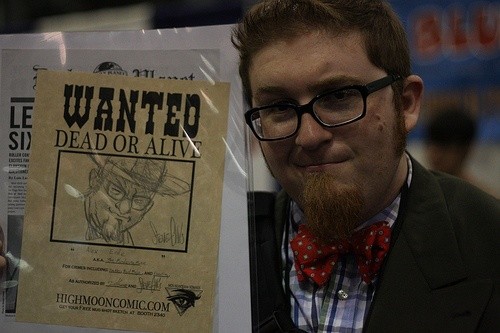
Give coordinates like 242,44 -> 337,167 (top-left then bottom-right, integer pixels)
0,0 -> 500,333
420,104 -> 482,189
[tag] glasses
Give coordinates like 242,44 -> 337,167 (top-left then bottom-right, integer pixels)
244,73 -> 404,141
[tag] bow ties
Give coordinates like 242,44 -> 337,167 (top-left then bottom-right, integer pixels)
290,220 -> 392,288
